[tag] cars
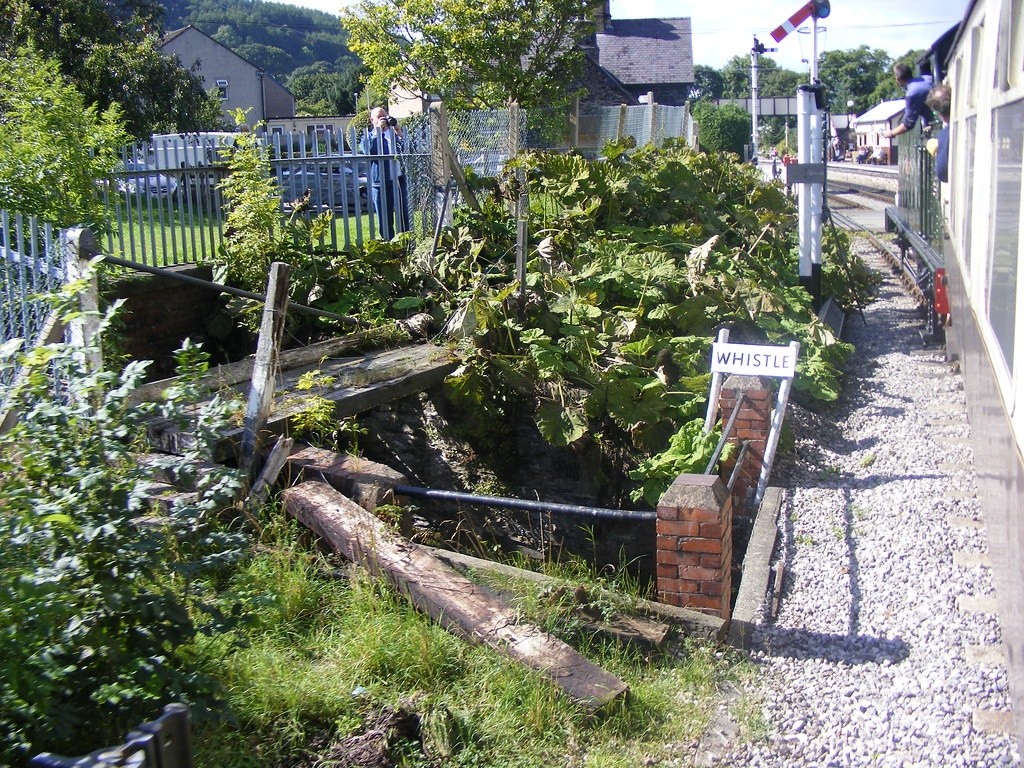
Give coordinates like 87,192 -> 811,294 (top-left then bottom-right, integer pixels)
95,159 -> 185,206
283,153 -> 369,208
471,155 -> 509,176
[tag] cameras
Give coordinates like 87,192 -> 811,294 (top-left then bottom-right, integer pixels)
383,116 -> 398,127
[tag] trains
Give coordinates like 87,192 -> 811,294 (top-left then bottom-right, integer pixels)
885,0 -> 1024,768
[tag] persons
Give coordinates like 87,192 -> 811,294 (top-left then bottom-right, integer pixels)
879,63 -> 933,139
855,145 -> 883,165
925,85 -> 952,183
360,107 -> 411,242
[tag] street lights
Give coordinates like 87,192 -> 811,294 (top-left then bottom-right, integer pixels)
353,93 -> 358,114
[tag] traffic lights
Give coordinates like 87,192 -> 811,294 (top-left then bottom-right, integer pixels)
812,0 -> 831,19
